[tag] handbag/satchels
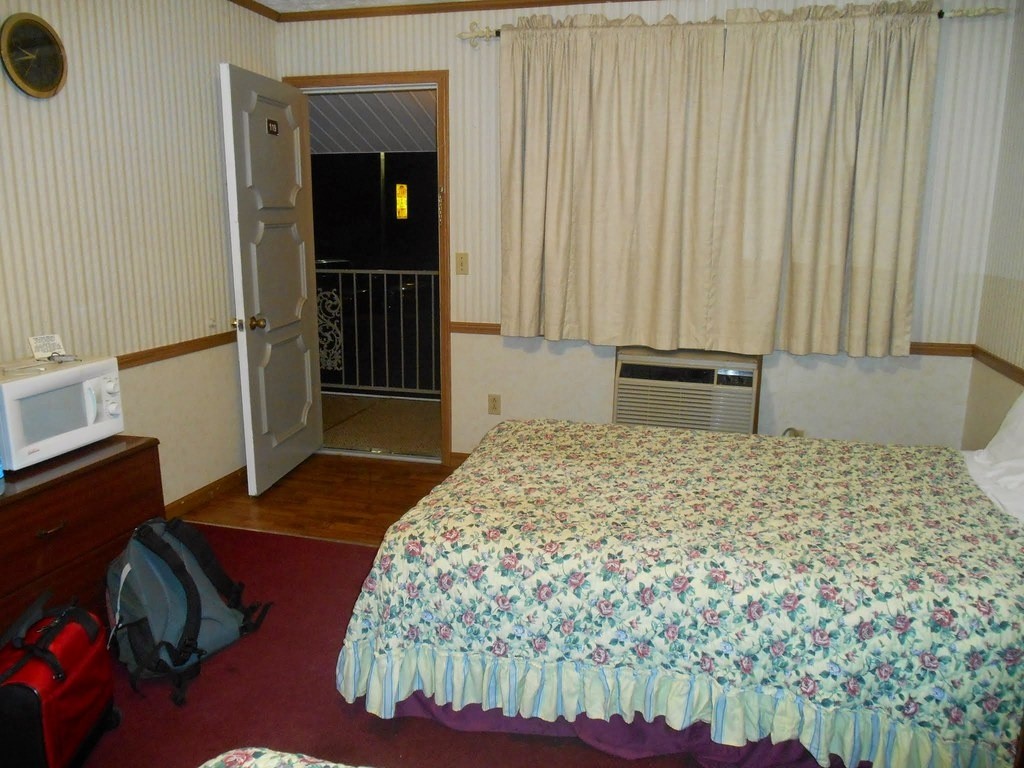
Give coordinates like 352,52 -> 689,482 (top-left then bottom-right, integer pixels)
1,591 -> 122,768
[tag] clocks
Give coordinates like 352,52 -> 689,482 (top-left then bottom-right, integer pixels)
1,11 -> 68,98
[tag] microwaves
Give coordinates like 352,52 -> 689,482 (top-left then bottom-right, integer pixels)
0,353 -> 125,474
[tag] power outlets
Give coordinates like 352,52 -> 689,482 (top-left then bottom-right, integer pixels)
487,393 -> 502,415
455,251 -> 469,275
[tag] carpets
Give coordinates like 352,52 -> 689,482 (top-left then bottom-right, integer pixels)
79,514 -> 690,767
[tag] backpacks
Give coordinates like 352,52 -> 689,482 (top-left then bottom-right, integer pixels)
106,516 -> 274,703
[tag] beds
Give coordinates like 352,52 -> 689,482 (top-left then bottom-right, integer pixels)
335,394 -> 1024,768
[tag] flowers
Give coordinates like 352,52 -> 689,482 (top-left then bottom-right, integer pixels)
298,750 -> 324,765
219,750 -> 252,766
361,416 -> 1024,760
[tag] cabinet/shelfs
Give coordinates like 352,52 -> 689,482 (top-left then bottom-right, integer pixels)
0,432 -> 167,638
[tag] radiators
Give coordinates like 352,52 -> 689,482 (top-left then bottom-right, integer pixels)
611,346 -> 760,434
